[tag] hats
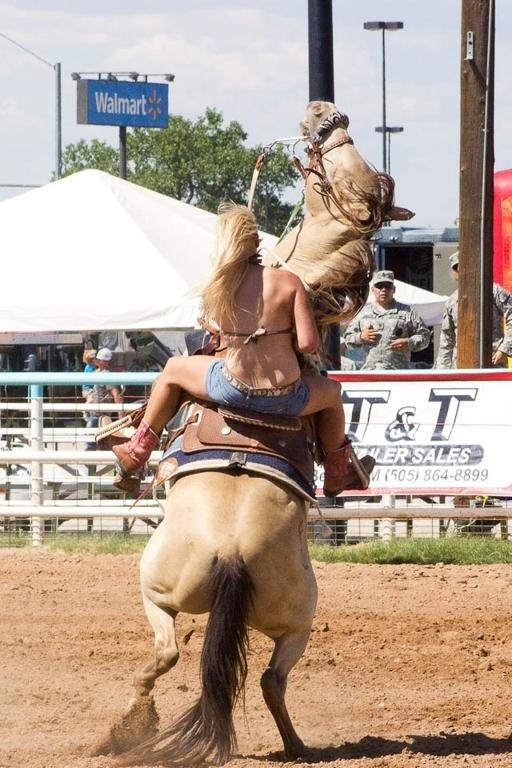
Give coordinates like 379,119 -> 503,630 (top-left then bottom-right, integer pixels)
373,271 -> 394,286
449,252 -> 459,268
96,348 -> 112,361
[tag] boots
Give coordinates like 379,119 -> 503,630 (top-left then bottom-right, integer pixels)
98,416 -> 159,480
317,436 -> 375,497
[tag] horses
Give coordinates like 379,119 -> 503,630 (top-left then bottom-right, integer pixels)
85,100 -> 415,767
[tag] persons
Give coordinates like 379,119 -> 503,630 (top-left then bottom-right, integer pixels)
344,270 -> 431,371
78,347 -> 123,429
433,251 -> 512,369
112,206 -> 375,495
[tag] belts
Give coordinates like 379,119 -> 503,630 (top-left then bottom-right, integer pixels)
222,367 -> 294,396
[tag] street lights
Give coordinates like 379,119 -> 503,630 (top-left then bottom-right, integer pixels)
363,19 -> 404,176
68,71 -> 175,180
373,125 -> 406,175
